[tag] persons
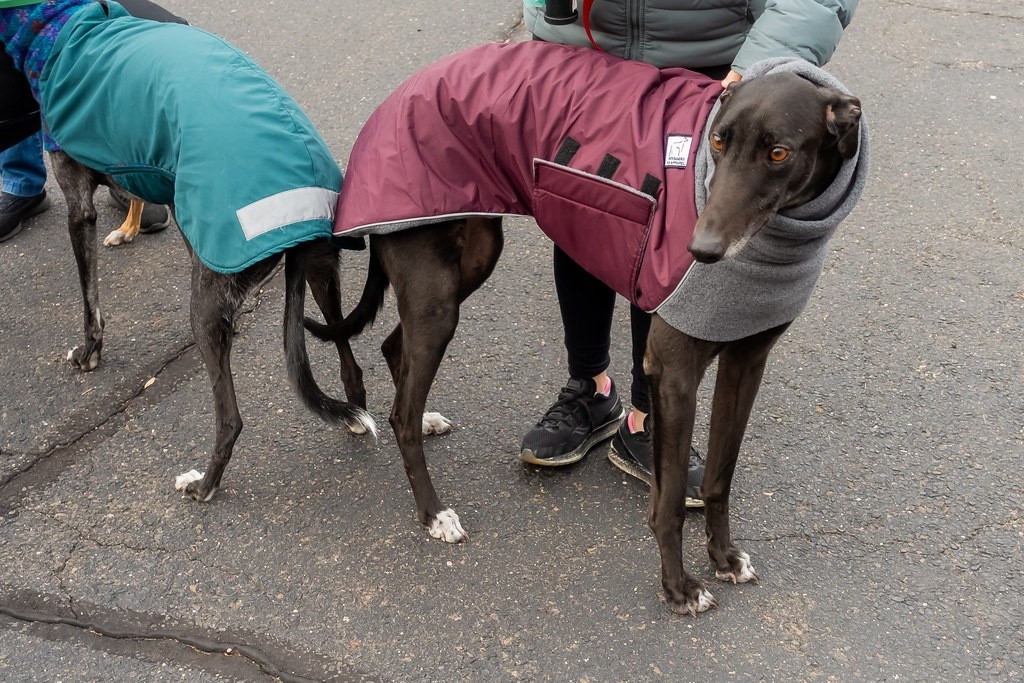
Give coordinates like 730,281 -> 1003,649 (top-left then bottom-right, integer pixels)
517,0 -> 860,507
0,0 -> 192,240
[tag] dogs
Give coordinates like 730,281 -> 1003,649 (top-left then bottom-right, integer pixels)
300,40 -> 865,620
1,0 -> 380,505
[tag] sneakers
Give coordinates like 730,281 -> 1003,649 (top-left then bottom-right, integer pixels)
608,412 -> 705,508
0,188 -> 50,242
108,188 -> 171,234
518,375 -> 626,465
